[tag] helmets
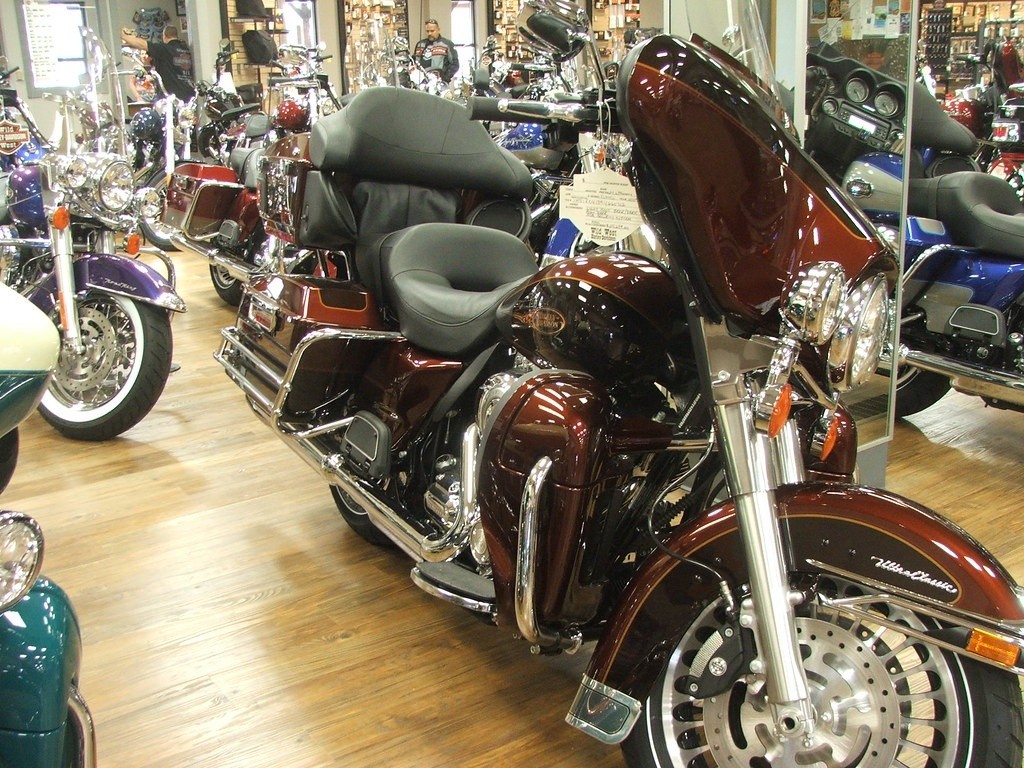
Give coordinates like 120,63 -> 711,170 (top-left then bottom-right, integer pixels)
130,108 -> 161,142
272,98 -> 311,129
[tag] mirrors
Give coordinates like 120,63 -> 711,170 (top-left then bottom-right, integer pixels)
797,0 -> 920,453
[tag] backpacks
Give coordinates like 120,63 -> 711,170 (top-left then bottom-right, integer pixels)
236,0 -> 269,18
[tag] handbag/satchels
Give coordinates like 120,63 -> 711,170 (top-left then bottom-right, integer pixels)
242,30 -> 278,63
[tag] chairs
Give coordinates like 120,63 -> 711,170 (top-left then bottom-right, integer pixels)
298,85 -> 534,362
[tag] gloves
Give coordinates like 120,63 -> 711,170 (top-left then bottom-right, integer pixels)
442,74 -> 452,83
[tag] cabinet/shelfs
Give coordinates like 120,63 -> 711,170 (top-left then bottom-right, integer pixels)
230,16 -> 283,75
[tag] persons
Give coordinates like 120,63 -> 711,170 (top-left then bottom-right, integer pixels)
121,25 -> 195,113
412,20 -> 459,89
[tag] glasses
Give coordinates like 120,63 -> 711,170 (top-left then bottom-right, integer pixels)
426,19 -> 437,23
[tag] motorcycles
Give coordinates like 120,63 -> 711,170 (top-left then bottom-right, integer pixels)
0,0 -> 1024,768
